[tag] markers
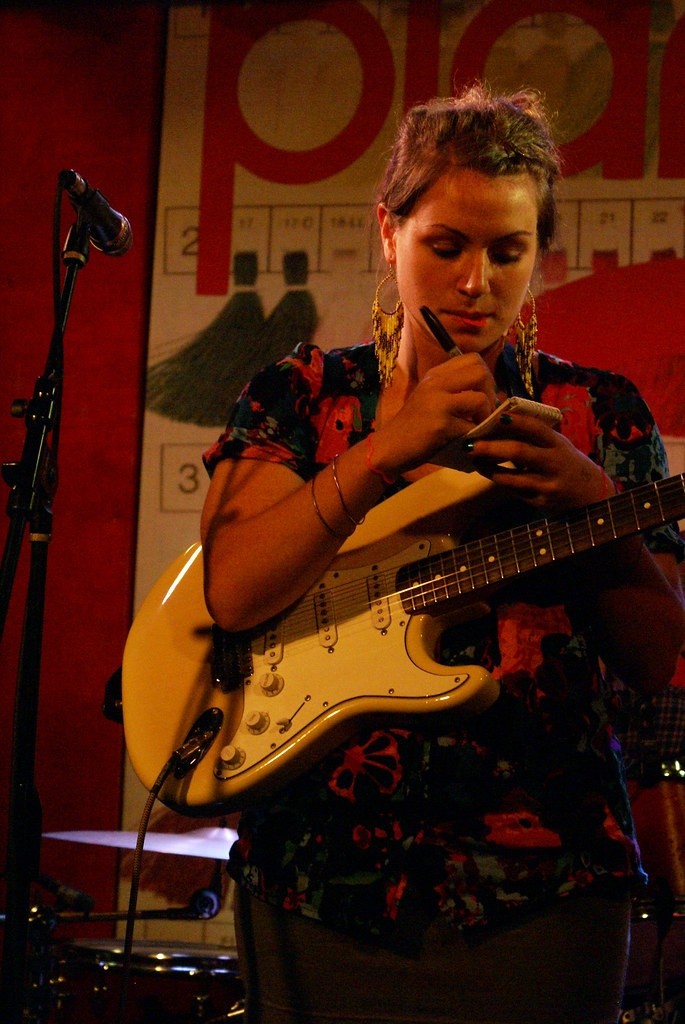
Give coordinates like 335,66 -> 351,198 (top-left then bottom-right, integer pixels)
418,306 -> 501,408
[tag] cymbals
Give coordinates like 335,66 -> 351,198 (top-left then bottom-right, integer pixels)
42,825 -> 240,860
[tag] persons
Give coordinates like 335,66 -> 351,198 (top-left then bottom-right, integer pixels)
200,80 -> 685,1024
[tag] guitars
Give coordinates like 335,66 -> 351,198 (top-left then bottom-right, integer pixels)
118,452 -> 685,820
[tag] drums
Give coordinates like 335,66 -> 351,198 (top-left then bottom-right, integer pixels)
621,755 -> 685,998
47,936 -> 247,1024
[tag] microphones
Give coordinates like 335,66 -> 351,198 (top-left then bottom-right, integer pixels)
60,170 -> 133,258
35,873 -> 95,913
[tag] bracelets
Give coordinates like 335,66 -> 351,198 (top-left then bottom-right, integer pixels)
332,454 -> 366,525
599,465 -> 609,500
310,471 -> 356,537
365,432 -> 399,486
631,536 -> 645,572
